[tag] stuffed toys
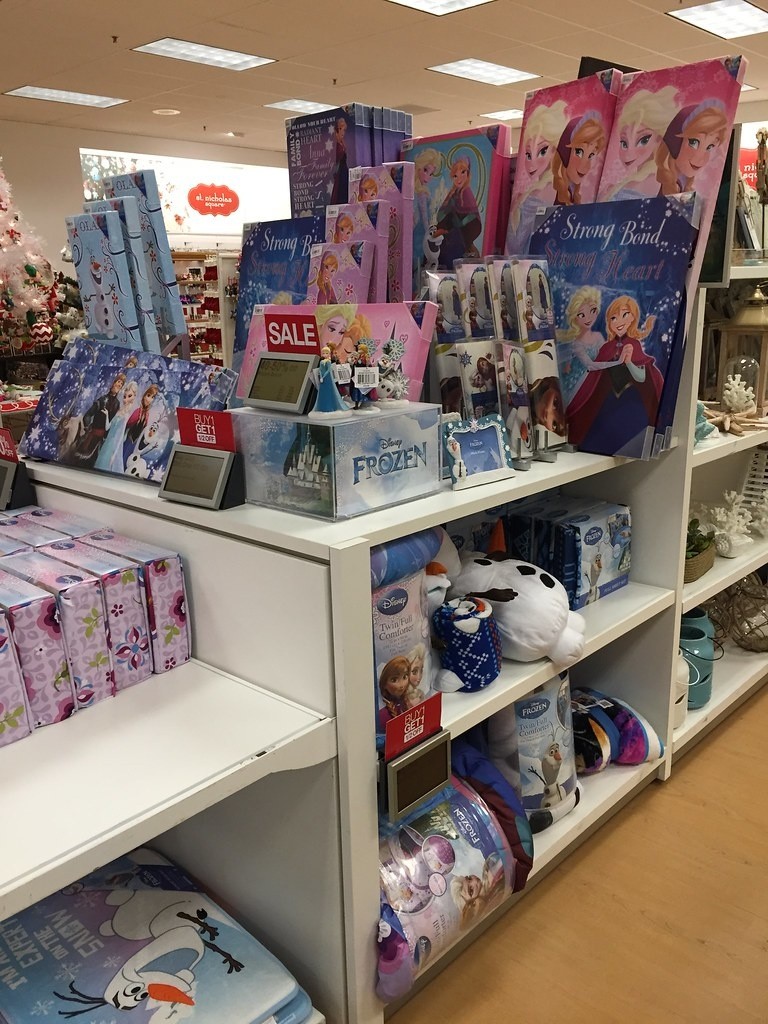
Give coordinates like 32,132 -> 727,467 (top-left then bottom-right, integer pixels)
424,526 -> 585,693
0,198 -> 59,351
570,686 -> 665,774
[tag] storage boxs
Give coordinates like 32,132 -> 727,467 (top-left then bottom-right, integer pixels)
12,53 -> 747,523
0,504 -> 192,747
443,489 -> 630,610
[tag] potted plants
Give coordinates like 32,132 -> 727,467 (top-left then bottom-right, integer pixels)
683,519 -> 717,583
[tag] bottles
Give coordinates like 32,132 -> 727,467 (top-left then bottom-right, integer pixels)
681,607 -> 714,650
679,624 -> 714,709
673,648 -> 690,729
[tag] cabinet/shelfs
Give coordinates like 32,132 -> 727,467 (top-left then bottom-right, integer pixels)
0,265 -> 768,1024
166,251 -> 241,370
